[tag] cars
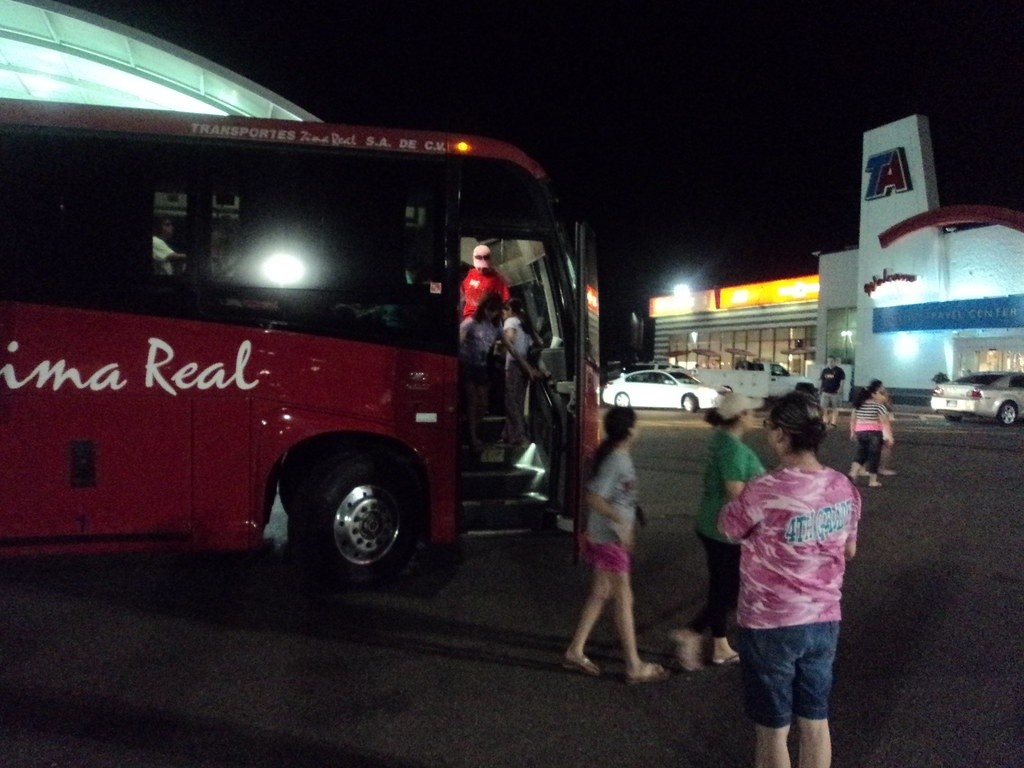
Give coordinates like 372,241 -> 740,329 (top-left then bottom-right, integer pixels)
634,362 -> 685,371
929,371 -> 1024,426
601,369 -> 732,412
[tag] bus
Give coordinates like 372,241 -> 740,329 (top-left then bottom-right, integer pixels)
1,95 -> 600,589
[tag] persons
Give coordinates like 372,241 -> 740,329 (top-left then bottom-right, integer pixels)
460,291 -> 535,445
672,393 -> 767,671
819,356 -> 846,428
153,217 -> 185,274
847,379 -> 896,486
562,406 -> 671,683
499,298 -> 553,445
460,245 -> 510,320
716,391 -> 861,768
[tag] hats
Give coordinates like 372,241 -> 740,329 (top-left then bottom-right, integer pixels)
717,394 -> 763,420
473,245 -> 492,269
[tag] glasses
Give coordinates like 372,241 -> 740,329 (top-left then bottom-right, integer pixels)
475,254 -> 491,261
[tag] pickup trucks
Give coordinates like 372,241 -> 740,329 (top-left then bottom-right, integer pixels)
694,361 -> 820,399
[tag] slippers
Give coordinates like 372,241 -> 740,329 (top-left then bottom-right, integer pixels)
626,664 -> 669,685
563,657 -> 599,676
668,628 -> 703,670
714,653 -> 739,666
